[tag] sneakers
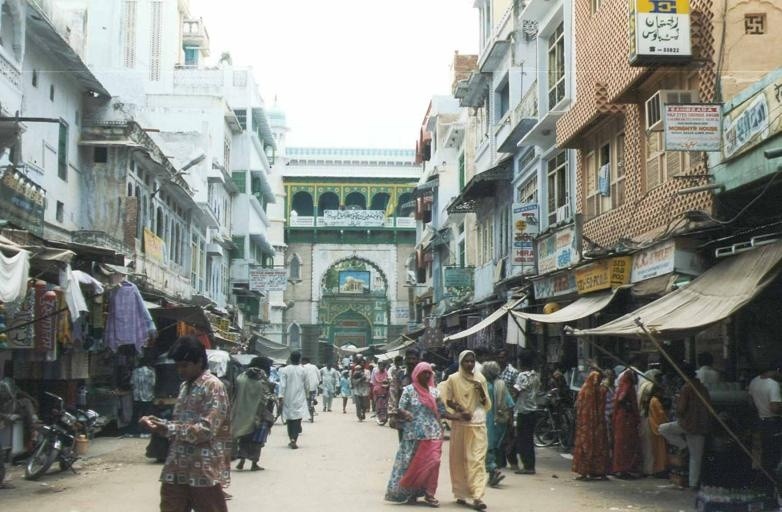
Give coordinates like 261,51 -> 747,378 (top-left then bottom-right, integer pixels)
400,464 -> 670,511
235,408 -> 367,471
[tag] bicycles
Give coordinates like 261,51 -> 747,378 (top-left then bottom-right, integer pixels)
306,391 -> 316,422
526,382 -> 576,450
372,382 -> 391,427
269,395 -> 287,426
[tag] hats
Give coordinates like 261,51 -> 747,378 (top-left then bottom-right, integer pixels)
168,335 -> 207,363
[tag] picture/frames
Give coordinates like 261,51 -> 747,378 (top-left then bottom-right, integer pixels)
339,271 -> 370,294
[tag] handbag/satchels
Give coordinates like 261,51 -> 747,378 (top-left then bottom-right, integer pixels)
493,379 -> 514,426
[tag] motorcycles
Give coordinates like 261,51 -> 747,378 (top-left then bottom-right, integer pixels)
21,389 -> 102,481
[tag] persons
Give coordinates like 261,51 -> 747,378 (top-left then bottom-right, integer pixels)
227,349 -> 320,472
125,359 -> 157,439
385,360 -> 445,508
445,349 -> 492,512
319,352 -> 457,423
476,349 -> 781,489
139,334 -> 232,512
